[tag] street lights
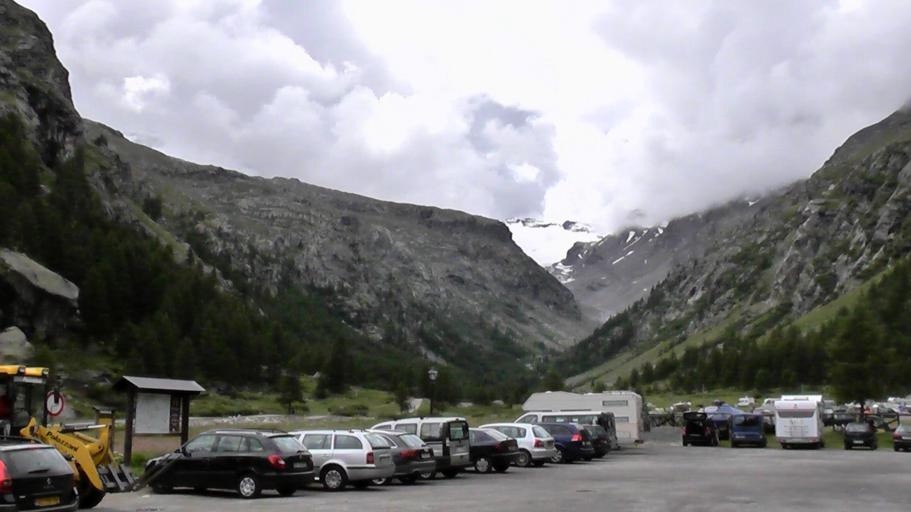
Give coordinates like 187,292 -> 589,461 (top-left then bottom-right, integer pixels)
425,367 -> 440,417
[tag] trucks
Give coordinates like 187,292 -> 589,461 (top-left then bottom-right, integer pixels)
519,390 -> 642,445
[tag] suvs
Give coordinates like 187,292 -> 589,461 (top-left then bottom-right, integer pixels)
0,434 -> 80,511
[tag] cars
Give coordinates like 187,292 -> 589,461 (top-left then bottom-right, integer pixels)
287,429 -> 395,492
682,394 -> 911,454
474,423 -> 555,469
449,427 -> 522,475
318,428 -> 437,488
534,421 -> 584,463
573,424 -> 612,462
146,428 -> 316,499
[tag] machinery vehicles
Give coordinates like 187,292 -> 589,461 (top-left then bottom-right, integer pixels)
0,364 -> 138,512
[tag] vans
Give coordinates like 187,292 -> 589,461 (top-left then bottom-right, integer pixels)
511,411 -> 619,448
370,415 -> 470,481
